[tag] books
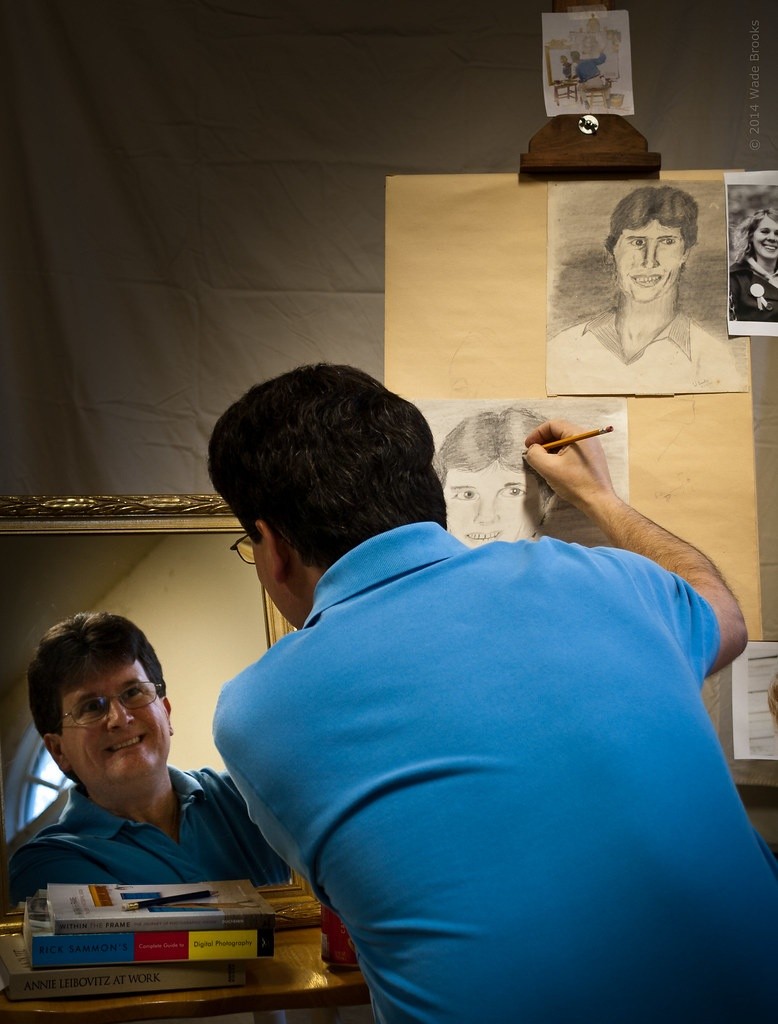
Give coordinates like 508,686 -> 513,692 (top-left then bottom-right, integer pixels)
0,880 -> 277,1002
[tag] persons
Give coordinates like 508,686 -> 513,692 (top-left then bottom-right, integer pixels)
9,611 -> 292,907
207,361 -> 778,1024
729,208 -> 778,322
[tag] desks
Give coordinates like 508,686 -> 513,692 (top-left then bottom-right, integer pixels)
0,926 -> 374,1024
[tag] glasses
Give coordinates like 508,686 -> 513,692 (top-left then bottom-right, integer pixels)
229,528 -> 256,564
61,681 -> 164,725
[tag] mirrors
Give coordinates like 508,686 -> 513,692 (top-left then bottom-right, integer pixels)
0,496 -> 322,936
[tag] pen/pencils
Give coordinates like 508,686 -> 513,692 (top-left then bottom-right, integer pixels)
521,426 -> 614,456
122,889 -> 218,911
